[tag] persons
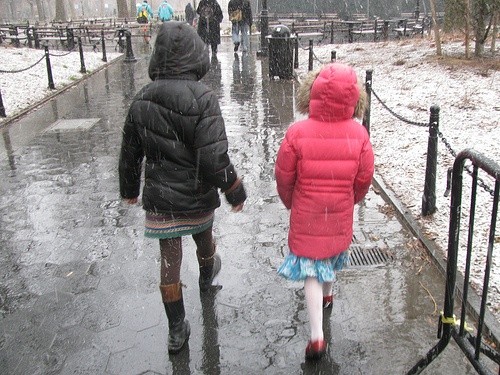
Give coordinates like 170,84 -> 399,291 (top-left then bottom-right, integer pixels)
185,3 -> 194,25
118,20 -> 248,353
227,0 -> 254,52
196,0 -> 223,53
136,0 -> 153,23
158,0 -> 174,21
275,61 -> 374,359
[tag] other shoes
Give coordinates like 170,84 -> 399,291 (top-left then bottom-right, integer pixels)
235,42 -> 238,52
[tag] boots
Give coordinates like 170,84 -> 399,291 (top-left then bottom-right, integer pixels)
196,245 -> 222,289
159,281 -> 190,351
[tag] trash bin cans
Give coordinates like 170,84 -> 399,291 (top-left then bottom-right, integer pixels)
266,33 -> 297,81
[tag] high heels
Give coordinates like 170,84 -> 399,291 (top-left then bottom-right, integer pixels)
323,296 -> 332,308
306,340 -> 327,369
